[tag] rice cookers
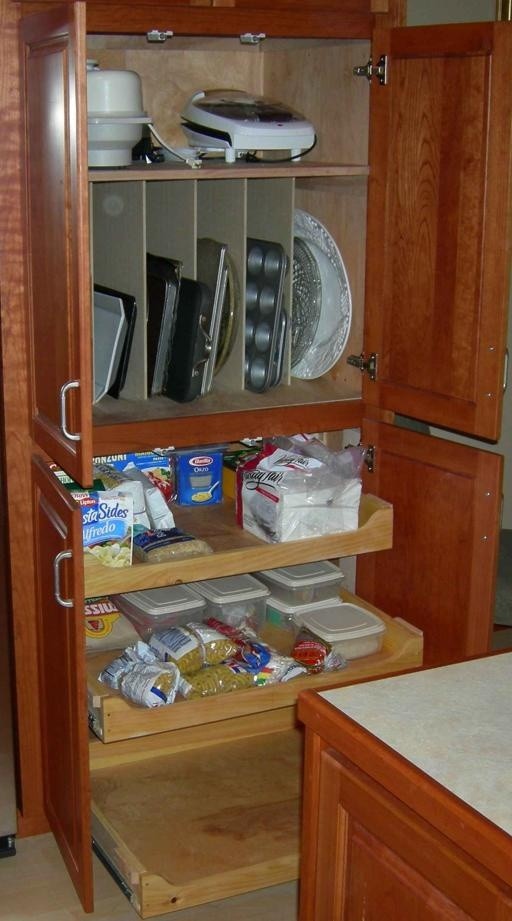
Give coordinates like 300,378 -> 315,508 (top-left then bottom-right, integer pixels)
87,58 -> 153,167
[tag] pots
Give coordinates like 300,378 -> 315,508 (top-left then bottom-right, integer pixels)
180,88 -> 315,163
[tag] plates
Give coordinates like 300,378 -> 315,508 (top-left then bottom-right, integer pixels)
291,207 -> 353,381
94,292 -> 128,406
214,253 -> 240,377
292,236 -> 322,368
95,283 -> 137,399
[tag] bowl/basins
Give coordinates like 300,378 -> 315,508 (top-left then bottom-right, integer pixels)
161,147 -> 197,162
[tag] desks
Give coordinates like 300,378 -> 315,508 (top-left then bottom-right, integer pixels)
296,648 -> 512,921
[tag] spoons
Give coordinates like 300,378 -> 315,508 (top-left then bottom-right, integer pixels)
191,480 -> 220,502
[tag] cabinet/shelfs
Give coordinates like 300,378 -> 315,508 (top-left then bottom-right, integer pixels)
0,417 -> 504,912
2,0 -> 512,489
